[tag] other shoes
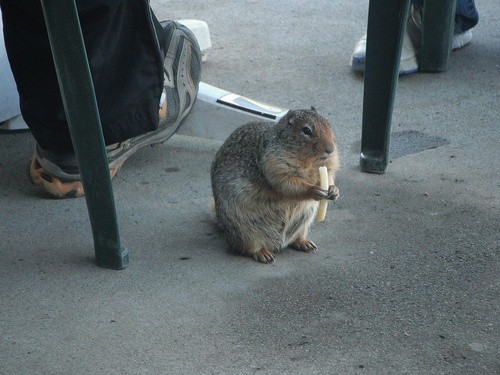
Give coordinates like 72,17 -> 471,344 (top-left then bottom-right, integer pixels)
350,29 -> 471,74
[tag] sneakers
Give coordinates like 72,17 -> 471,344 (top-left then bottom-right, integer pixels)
31,17 -> 202,198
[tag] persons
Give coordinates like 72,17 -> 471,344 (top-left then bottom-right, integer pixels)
349,0 -> 481,82
0,0 -> 203,200
